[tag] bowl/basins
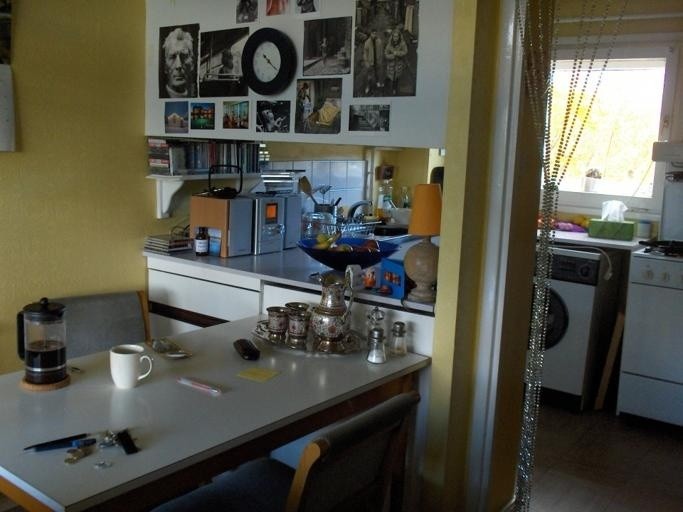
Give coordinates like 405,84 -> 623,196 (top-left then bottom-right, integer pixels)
389,207 -> 412,224
295,237 -> 399,272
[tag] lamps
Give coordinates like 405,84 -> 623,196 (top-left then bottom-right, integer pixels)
404,184 -> 441,304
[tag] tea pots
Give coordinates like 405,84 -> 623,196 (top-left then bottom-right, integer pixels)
308,269 -> 354,354
16,296 -> 69,385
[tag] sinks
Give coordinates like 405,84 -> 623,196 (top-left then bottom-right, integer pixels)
353,227 -> 407,237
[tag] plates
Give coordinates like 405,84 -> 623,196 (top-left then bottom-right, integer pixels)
251,319 -> 368,359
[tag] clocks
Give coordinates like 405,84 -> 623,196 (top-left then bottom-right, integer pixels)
242,27 -> 297,95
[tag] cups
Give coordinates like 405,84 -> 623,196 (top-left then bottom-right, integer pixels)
284,302 -> 311,352
266,306 -> 287,343
109,343 -> 153,390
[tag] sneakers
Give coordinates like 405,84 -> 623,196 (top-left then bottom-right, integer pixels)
381,83 -> 385,87
365,87 -> 369,93
376,82 -> 384,87
392,88 -> 397,94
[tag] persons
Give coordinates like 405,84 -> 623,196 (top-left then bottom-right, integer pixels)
320,38 -> 329,67
161,28 -> 196,98
362,29 -> 380,94
384,29 -> 409,94
295,83 -> 312,129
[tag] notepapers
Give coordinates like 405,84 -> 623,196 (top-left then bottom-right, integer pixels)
237,367 -> 281,386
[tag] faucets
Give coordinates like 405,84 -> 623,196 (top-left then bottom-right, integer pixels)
346,200 -> 372,219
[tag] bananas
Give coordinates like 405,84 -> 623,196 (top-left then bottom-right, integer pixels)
312,233 -> 340,249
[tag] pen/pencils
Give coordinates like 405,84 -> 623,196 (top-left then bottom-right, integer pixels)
23,433 -> 97,454
177,378 -> 220,395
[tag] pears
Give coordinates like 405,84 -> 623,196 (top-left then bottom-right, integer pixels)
316,233 -> 330,242
337,244 -> 353,253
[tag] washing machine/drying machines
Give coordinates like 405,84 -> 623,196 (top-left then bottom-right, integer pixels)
522,242 -> 624,408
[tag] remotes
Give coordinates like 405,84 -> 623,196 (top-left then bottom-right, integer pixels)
234,338 -> 260,360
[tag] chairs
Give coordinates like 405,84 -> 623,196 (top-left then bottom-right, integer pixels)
152,391 -> 422,512
46,289 -> 153,359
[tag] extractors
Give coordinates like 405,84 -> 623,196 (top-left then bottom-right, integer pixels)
652,141 -> 683,184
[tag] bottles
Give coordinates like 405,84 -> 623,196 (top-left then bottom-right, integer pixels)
390,321 -> 407,358
400,185 -> 411,208
367,327 -> 387,364
377,178 -> 393,218
194,226 -> 211,256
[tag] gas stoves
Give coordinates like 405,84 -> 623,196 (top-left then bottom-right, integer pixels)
638,238 -> 683,256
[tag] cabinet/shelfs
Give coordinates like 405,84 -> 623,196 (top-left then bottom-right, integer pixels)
141,244 -> 434,481
